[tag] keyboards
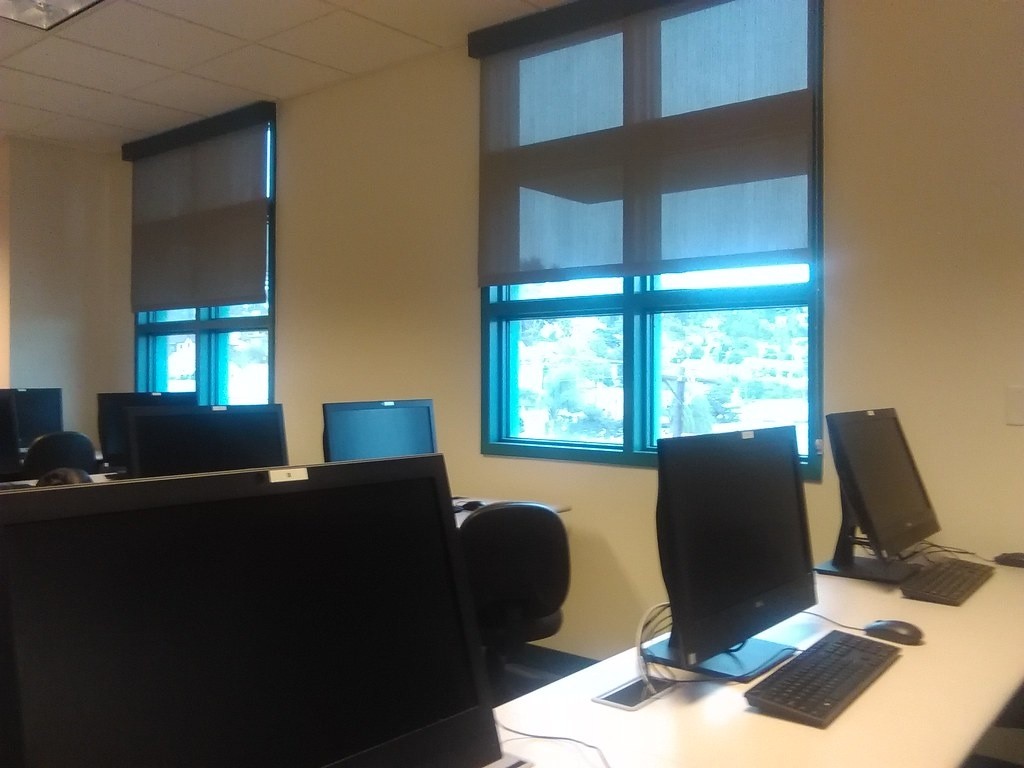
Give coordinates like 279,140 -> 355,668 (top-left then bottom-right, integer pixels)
900,556 -> 996,606
744,629 -> 901,730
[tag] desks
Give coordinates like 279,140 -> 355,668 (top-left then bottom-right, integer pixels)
11,472 -> 117,486
19,447 -> 103,465
492,549 -> 1024,768
452,498 -> 573,528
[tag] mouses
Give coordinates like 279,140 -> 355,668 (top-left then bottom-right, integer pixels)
864,620 -> 922,645
994,552 -> 1024,568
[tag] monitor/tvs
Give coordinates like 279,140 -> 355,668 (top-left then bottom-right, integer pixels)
814,408 -> 943,584
641,423 -> 818,684
0,387 -> 502,768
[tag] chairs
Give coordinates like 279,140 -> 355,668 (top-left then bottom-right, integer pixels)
459,501 -> 571,706
19,431 -> 97,479
